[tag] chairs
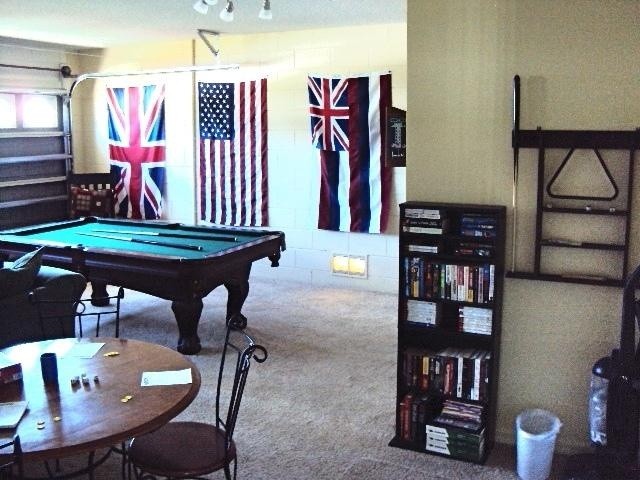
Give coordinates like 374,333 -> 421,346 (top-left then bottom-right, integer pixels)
127,314 -> 268,479
0,434 -> 25,480
67,165 -> 114,221
29,287 -> 125,341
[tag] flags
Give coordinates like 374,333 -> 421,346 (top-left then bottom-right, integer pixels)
317,70 -> 394,236
104,85 -> 167,219
197,78 -> 268,227
307,76 -> 351,153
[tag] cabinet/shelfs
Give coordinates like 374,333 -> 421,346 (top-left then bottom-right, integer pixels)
388,200 -> 507,467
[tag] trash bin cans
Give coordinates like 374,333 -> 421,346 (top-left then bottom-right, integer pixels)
516,408 -> 560,480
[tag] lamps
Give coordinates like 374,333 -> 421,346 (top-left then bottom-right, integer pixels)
193,0 -> 272,22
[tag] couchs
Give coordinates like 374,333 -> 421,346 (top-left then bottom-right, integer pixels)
2,261 -> 86,350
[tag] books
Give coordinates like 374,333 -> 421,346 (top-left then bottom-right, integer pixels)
1,400 -> 29,429
394,204 -> 498,462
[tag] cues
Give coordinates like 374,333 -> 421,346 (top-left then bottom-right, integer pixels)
511,75 -> 521,275
94,230 -> 237,243
74,232 -> 203,252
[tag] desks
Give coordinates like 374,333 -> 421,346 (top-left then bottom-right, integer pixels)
0,337 -> 201,480
0,216 -> 287,355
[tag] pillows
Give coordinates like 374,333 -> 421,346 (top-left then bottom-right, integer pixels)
0,246 -> 44,298
69,186 -> 112,218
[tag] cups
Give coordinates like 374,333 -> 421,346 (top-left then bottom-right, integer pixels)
40,352 -> 58,385
